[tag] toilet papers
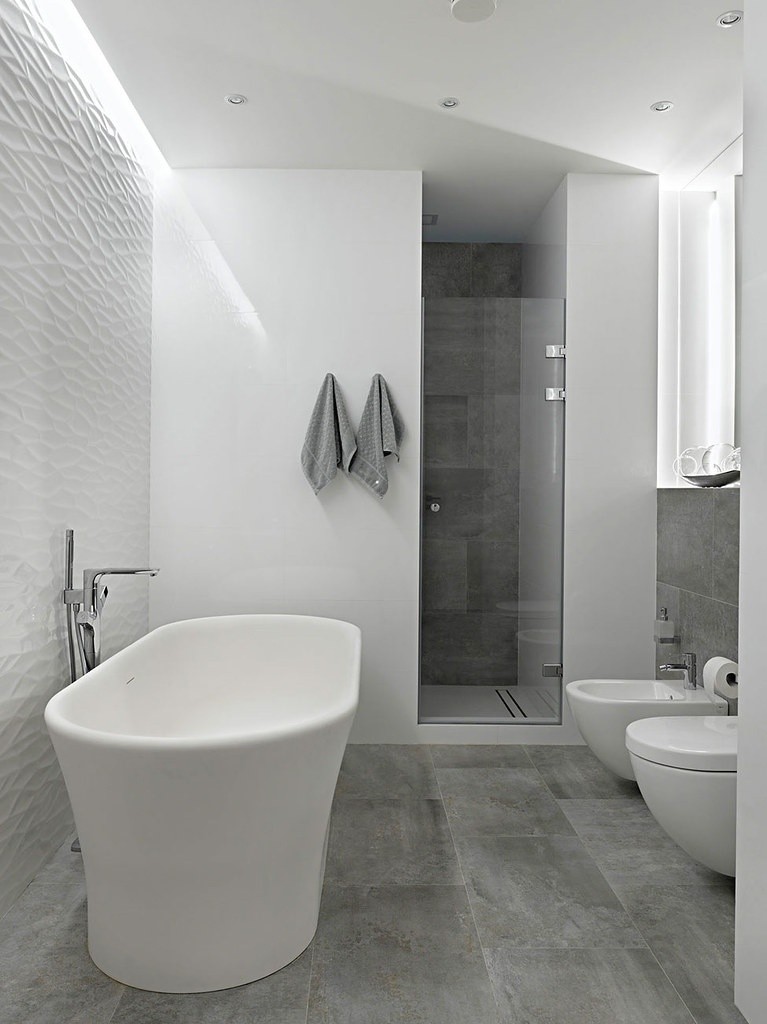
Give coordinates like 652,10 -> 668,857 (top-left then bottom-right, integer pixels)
702,656 -> 738,713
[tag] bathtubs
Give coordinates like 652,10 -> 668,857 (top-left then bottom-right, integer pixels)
40,611 -> 364,996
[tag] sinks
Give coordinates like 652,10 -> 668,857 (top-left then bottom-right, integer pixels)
564,679 -> 729,783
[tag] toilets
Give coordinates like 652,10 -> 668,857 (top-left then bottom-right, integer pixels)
624,715 -> 737,879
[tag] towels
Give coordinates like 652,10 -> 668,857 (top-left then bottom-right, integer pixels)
347,372 -> 407,502
298,372 -> 358,497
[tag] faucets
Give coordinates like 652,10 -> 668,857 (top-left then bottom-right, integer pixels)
75,567 -> 161,674
657,650 -> 697,691
